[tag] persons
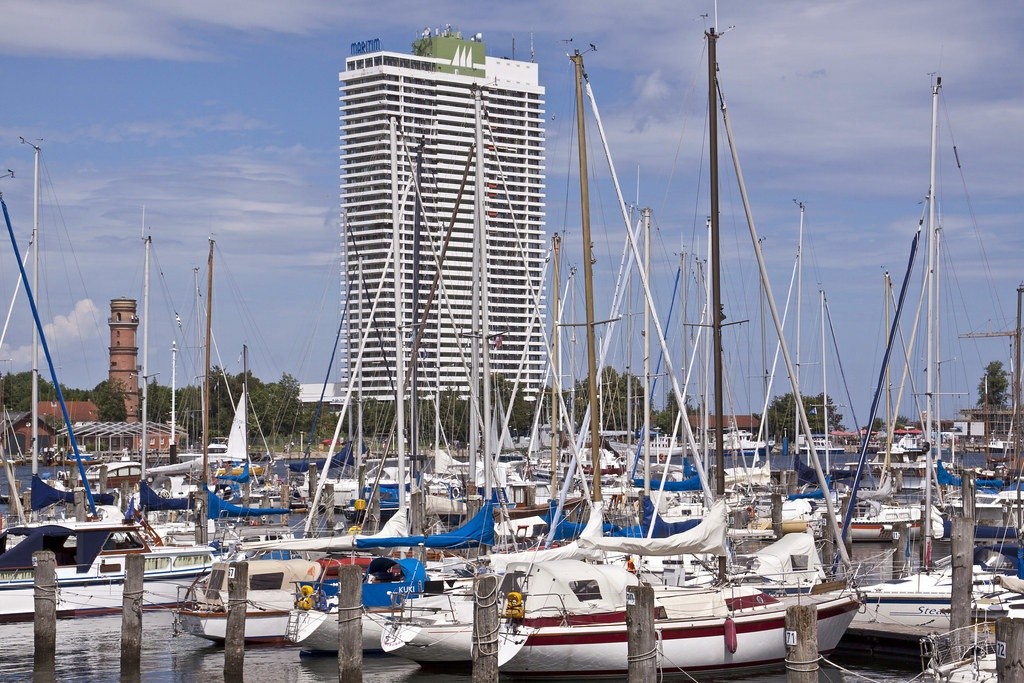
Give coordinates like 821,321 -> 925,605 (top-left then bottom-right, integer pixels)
626,557 -> 636,574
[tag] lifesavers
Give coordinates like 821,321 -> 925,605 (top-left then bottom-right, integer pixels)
451,487 -> 460,500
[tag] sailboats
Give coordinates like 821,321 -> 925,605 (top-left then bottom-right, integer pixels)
285,82 -> 585,654
25,138 -> 1020,544
2,164 -> 237,616
181,128 -> 468,642
823,75 -> 1024,658
380,35 -> 869,675
